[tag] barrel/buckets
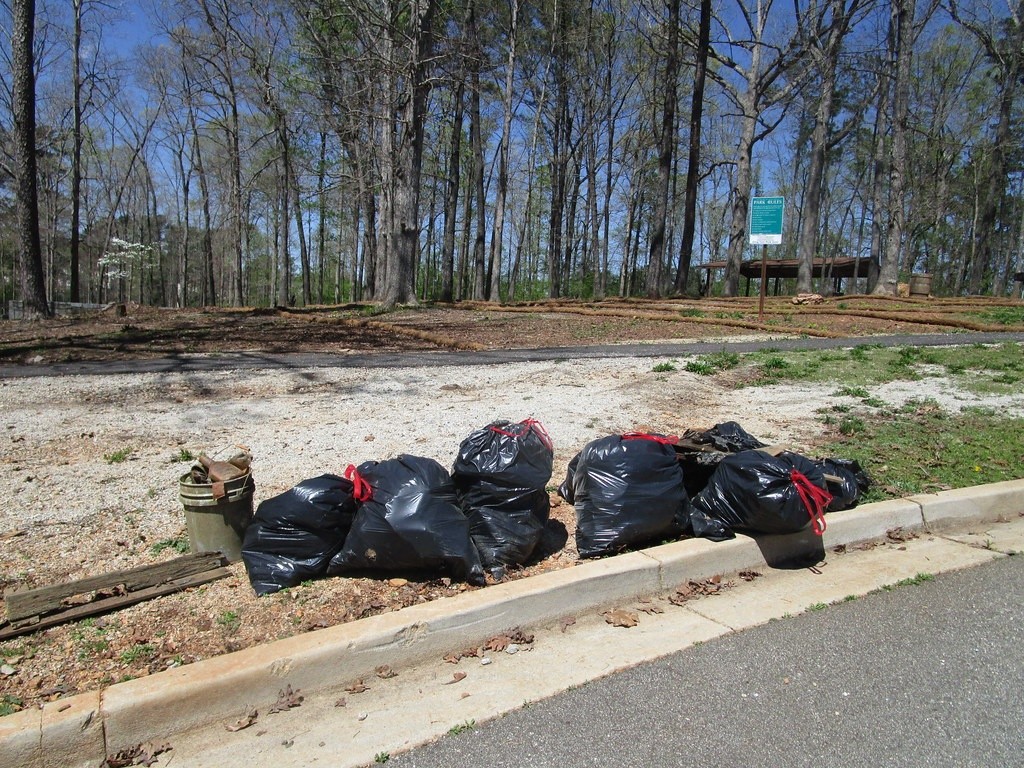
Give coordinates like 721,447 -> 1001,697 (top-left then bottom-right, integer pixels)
909,273 -> 933,298
179,442 -> 255,563
